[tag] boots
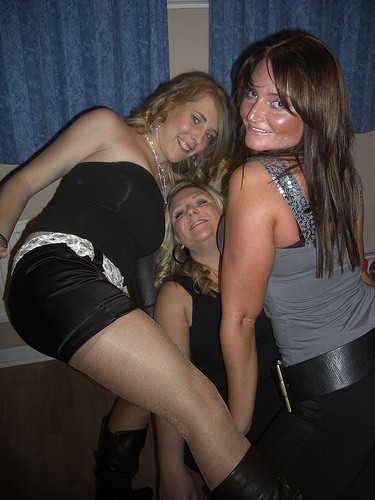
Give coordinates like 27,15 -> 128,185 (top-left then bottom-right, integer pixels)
95,415 -> 152,500
204,444 -> 310,500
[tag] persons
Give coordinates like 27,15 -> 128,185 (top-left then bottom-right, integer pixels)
0,71 -> 306,499
151,179 -> 294,500
219,30 -> 374,499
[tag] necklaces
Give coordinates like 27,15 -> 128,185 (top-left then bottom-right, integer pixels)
144,132 -> 167,204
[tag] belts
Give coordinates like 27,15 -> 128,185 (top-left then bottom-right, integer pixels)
264,328 -> 372,412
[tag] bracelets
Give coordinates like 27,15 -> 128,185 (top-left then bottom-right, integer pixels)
0,234 -> 9,248
